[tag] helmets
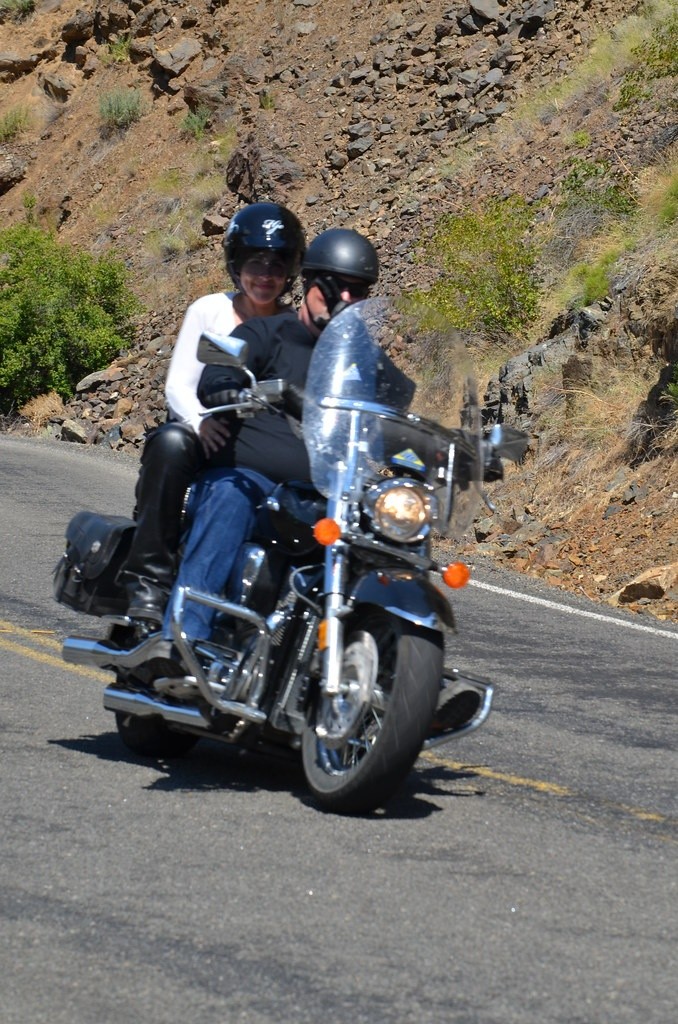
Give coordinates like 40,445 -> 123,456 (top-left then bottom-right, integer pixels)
300,228 -> 380,303
220,202 -> 306,288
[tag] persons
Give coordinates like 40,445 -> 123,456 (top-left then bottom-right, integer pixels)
97,203 -> 319,652
141,226 -> 483,744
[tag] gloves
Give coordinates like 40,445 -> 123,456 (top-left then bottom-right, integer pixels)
202,376 -> 242,408
433,428 -> 504,492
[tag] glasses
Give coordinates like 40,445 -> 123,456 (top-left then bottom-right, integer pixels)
310,273 -> 370,299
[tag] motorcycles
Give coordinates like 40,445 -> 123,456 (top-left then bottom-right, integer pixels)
65,296 -> 532,812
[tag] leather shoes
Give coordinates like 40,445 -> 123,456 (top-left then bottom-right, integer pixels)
149,645 -> 198,707
425,679 -> 481,740
126,599 -> 163,625
